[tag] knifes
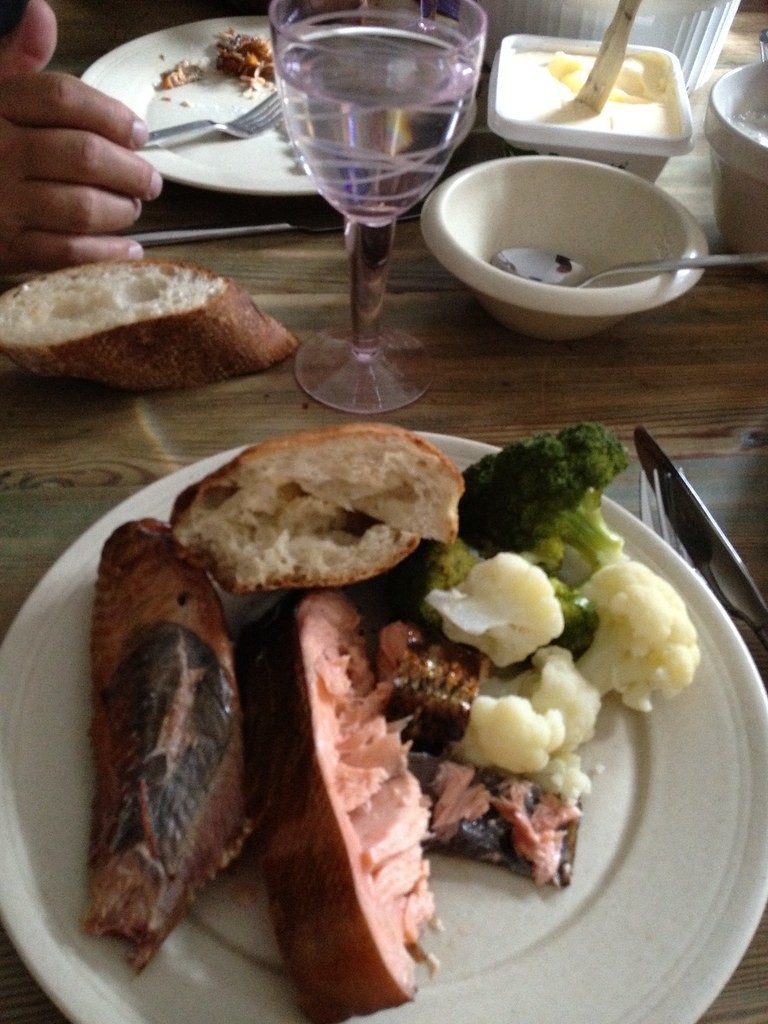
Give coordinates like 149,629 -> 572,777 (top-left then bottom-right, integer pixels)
633,426 -> 768,652
117,213 -> 428,245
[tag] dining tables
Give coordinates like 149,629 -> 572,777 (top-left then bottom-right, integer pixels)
0,0 -> 768,1024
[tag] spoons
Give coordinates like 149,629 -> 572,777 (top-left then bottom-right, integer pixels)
491,248 -> 768,289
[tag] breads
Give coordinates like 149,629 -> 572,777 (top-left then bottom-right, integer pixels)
170,423 -> 464,590
1,264 -> 296,389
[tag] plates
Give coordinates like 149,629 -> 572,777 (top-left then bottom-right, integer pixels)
79,15 -> 323,196
0,431 -> 768,1024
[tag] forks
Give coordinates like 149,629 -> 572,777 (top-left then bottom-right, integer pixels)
640,467 -> 696,568
145,91 -> 284,148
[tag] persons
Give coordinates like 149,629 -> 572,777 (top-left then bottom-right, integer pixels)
0,0 -> 164,273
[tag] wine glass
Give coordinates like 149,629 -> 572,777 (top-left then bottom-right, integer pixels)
269,0 -> 487,414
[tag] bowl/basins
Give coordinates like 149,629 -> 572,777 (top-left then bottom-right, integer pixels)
703,61 -> 768,254
420,156 -> 710,342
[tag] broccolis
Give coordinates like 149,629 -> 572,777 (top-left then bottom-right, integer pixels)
428,424 -> 697,793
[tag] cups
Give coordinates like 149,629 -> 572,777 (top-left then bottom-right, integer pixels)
487,33 -> 695,185
